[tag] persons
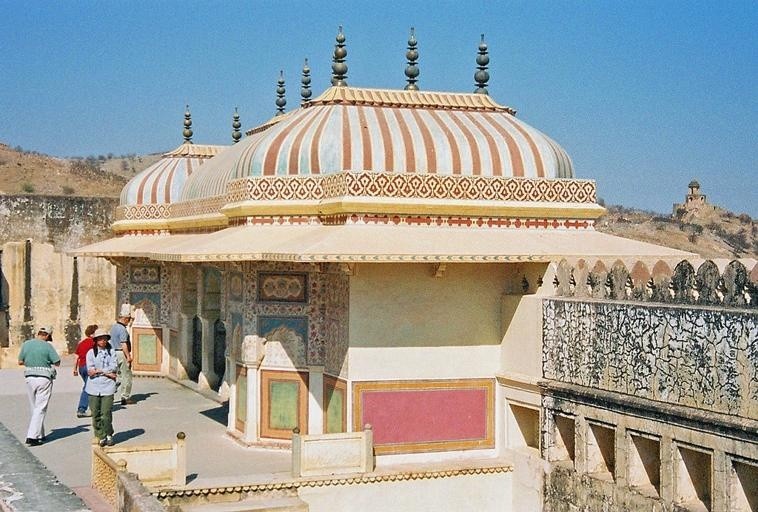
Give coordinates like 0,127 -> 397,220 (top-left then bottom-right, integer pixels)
17,325 -> 60,446
110,310 -> 134,405
84,328 -> 118,447
73,325 -> 98,418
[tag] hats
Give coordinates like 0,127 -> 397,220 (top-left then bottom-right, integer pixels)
118,308 -> 131,318
39,325 -> 53,341
90,329 -> 111,341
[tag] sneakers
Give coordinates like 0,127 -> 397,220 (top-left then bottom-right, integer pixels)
76,412 -> 90,418
98,437 -> 113,447
122,399 -> 136,404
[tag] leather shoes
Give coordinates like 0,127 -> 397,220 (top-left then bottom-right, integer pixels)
26,438 -> 47,446
